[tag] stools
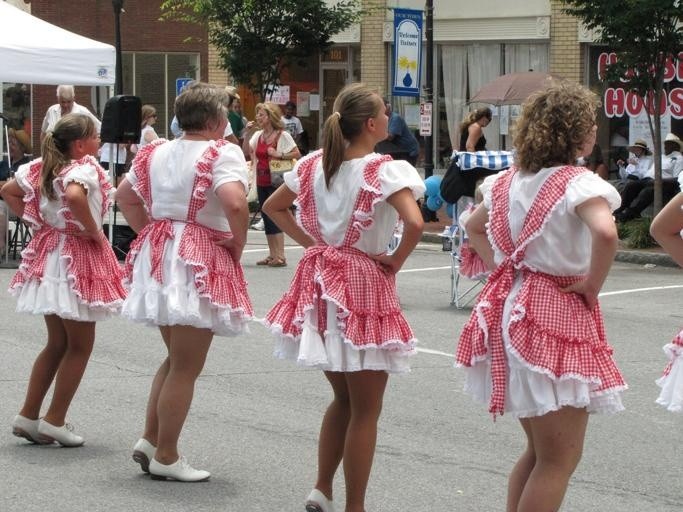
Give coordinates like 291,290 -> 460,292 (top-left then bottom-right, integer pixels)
7,216 -> 35,262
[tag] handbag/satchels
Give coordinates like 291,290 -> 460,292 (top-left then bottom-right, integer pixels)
268,156 -> 298,188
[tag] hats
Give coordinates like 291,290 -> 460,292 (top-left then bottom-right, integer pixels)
662,133 -> 683,153
223,86 -> 241,99
626,139 -> 652,155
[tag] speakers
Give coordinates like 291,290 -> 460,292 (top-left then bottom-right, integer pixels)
101,95 -> 141,144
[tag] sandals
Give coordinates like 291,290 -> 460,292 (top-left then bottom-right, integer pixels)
268,256 -> 287,267
257,257 -> 272,265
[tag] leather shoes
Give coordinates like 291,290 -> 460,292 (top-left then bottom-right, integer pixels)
132,438 -> 212,483
12,414 -> 55,445
306,488 -> 337,512
37,417 -> 86,447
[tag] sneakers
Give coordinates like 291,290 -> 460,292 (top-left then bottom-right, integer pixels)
251,217 -> 265,231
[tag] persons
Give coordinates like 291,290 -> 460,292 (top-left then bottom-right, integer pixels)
0,113 -> 130,447
261,82 -> 427,512
2,80 -> 304,267
113,79 -> 251,482
454,82 -> 630,512
649,170 -> 683,414
581,132 -> 683,224
417,107 -> 491,231
373,100 -> 420,167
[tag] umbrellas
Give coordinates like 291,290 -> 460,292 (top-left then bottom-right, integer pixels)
466,68 -> 568,107
